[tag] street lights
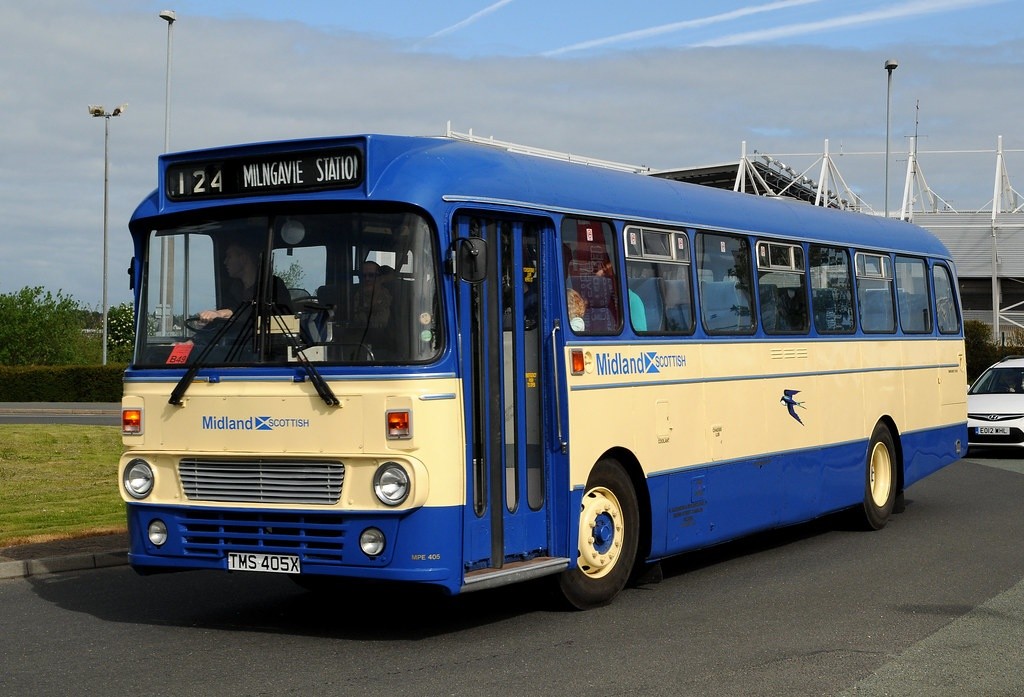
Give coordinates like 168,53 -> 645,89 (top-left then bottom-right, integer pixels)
159,8 -> 177,338
884,57 -> 899,217
87,102 -> 125,367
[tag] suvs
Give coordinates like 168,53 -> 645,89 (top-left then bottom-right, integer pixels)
965,355 -> 1024,457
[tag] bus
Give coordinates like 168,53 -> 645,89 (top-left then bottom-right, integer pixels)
116,120 -> 968,612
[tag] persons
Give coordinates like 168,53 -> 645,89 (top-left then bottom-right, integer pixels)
192,235 -> 294,347
341,258 -> 393,328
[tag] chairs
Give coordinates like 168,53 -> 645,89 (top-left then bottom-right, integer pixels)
759,284 -> 780,333
566,276 -> 621,334
839,289 -> 865,333
866,290 -> 889,330
910,294 -> 926,330
630,277 -> 666,332
776,287 -> 806,333
702,282 -> 743,332
889,292 -> 911,330
665,279 -> 692,333
812,288 -> 839,332
